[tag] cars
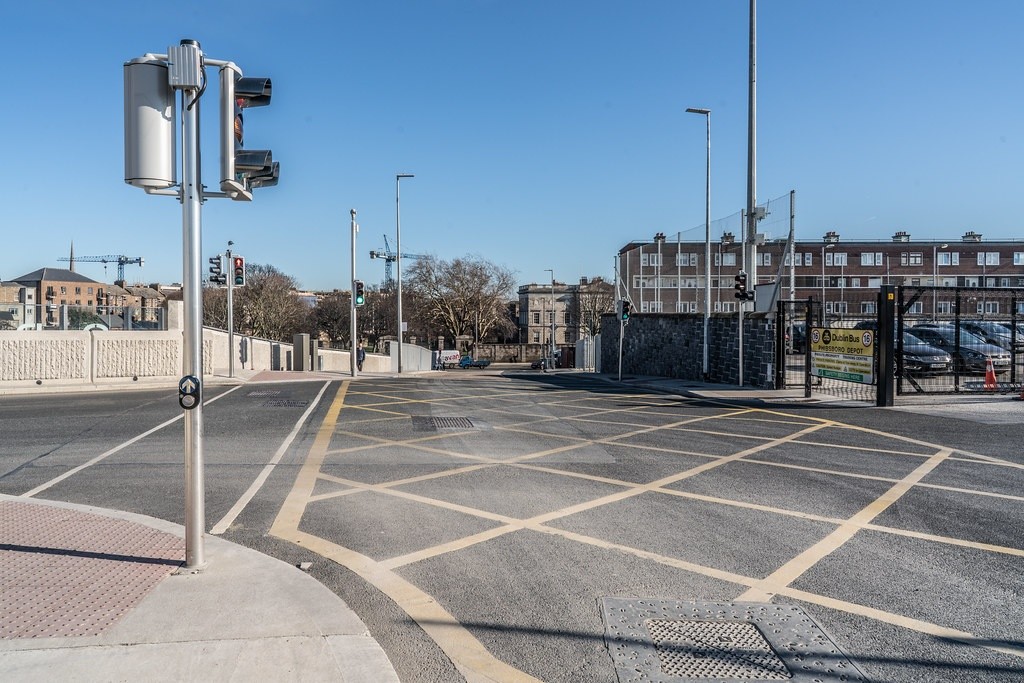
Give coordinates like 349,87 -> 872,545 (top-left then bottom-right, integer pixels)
875,330 -> 952,376
904,327 -> 1011,376
532,358 -> 558,369
852,319 -> 1024,350
786,323 -> 816,354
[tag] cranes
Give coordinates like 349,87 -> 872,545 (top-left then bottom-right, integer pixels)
56,255 -> 144,282
369,234 -> 432,291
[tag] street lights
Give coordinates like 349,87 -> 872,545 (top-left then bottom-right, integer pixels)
686,107 -> 711,374
396,173 -> 414,373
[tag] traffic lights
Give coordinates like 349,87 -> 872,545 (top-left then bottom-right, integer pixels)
233,70 -> 272,189
233,257 -> 245,287
745,292 -> 754,301
354,281 -> 365,305
215,274 -> 227,286
209,255 -> 221,285
735,274 -> 747,299
622,300 -> 630,321
245,161 -> 280,196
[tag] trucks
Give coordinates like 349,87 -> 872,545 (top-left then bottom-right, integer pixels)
434,350 -> 460,369
459,356 -> 490,369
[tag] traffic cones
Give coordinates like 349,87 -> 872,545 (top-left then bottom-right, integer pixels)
985,355 -> 999,389
1020,381 -> 1024,400
